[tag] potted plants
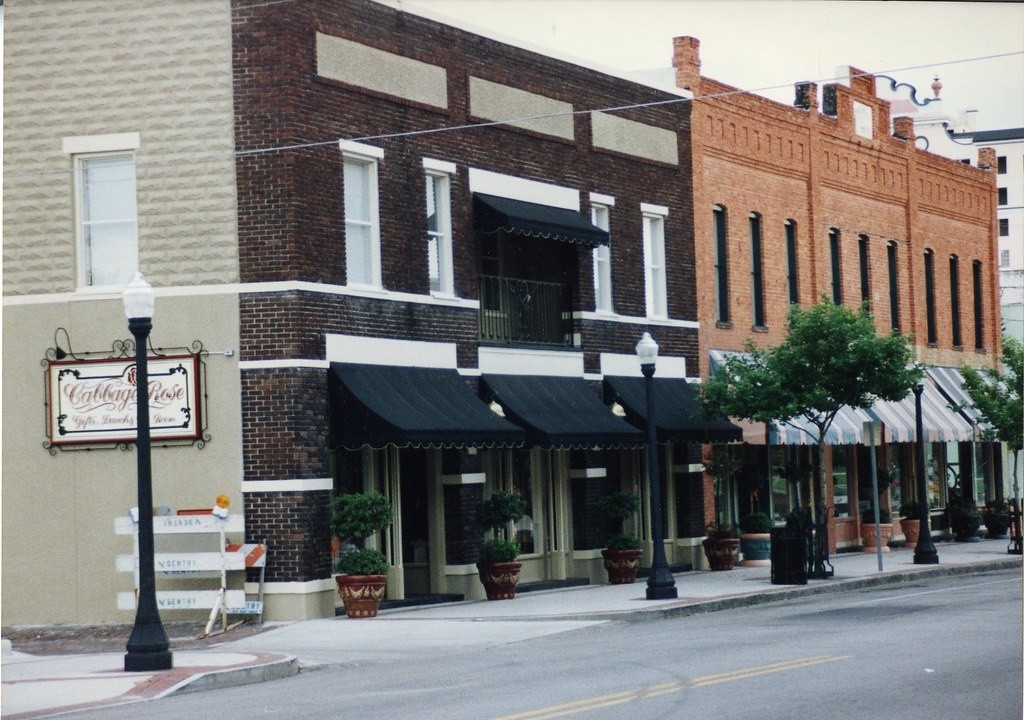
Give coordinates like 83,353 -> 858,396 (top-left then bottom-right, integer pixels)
897,499 -> 936,542
702,442 -> 741,570
949,498 -> 981,535
982,495 -> 1011,534
736,512 -> 774,560
326,490 -> 396,618
860,508 -> 894,546
473,490 -> 528,600
593,490 -> 643,584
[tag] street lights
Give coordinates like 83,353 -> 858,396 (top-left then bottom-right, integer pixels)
912,360 -> 940,564
121,269 -> 174,672
634,331 -> 679,599
